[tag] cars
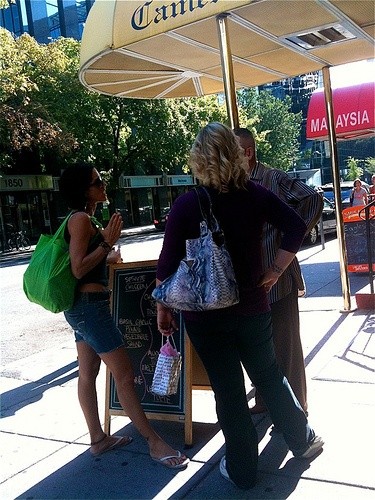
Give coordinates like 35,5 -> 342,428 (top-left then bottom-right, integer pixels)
304,180 -> 375,245
153,207 -> 172,232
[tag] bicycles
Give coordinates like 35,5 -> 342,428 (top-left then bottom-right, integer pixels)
7,230 -> 31,252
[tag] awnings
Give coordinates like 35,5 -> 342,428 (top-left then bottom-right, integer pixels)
369,174 -> 375,204
350,180 -> 368,205
305,81 -> 375,140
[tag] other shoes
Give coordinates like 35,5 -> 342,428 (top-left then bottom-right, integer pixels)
272,411 -> 308,432
250,402 -> 267,414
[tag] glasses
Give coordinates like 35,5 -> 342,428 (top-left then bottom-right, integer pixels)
89,175 -> 104,189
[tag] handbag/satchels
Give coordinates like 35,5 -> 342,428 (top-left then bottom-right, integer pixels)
151,185 -> 240,310
22,210 -> 92,313
151,333 -> 182,396
350,189 -> 355,204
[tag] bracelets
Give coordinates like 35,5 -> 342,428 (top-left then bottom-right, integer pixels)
272,265 -> 284,274
99,241 -> 112,254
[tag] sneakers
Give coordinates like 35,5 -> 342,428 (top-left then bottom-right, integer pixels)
298,433 -> 325,459
219,455 -> 239,489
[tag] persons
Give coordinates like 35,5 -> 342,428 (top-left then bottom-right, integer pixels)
51,160 -> 192,467
231,127 -> 324,433
155,120 -> 326,491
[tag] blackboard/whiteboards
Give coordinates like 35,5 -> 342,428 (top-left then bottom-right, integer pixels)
106,259 -> 191,423
343,219 -> 375,264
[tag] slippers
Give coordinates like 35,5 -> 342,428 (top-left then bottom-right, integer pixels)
152,450 -> 190,468
90,436 -> 132,456
